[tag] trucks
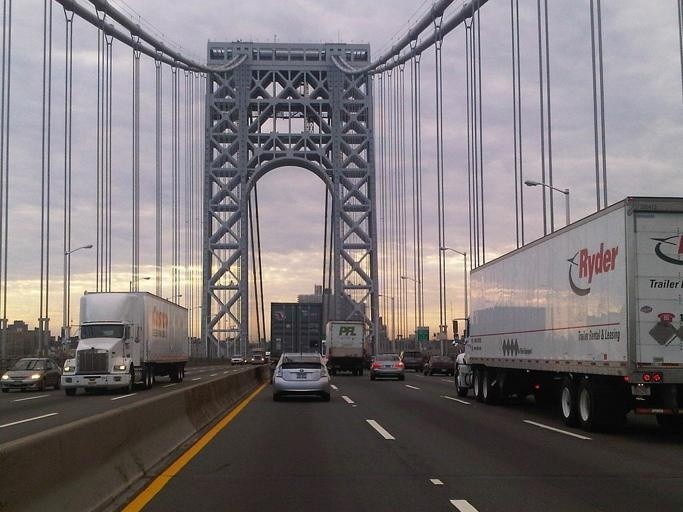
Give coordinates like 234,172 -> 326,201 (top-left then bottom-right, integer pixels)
325,320 -> 366,375
251,348 -> 264,365
453,196 -> 683,434
60,292 -> 189,396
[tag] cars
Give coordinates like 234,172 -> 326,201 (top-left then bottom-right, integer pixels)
370,352 -> 405,380
0,358 -> 62,392
272,352 -> 330,401
400,350 -> 453,376
230,354 -> 244,366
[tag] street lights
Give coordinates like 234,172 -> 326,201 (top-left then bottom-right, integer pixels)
167,295 -> 182,301
130,277 -> 150,292
64,245 -> 93,326
524,180 -> 570,226
439,247 -> 468,329
401,275 -> 420,324
378,294 -> 395,353
